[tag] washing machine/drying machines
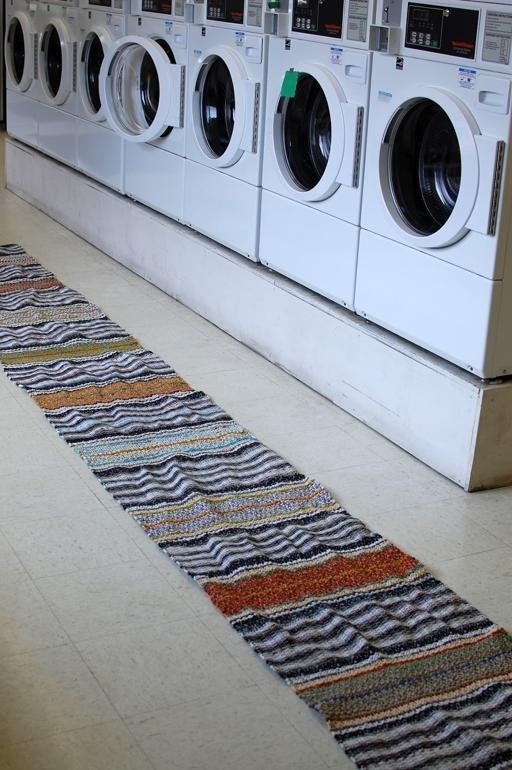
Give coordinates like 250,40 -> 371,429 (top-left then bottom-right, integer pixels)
3,0 -> 512,381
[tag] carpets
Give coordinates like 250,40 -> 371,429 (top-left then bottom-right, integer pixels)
3,239 -> 512,769
